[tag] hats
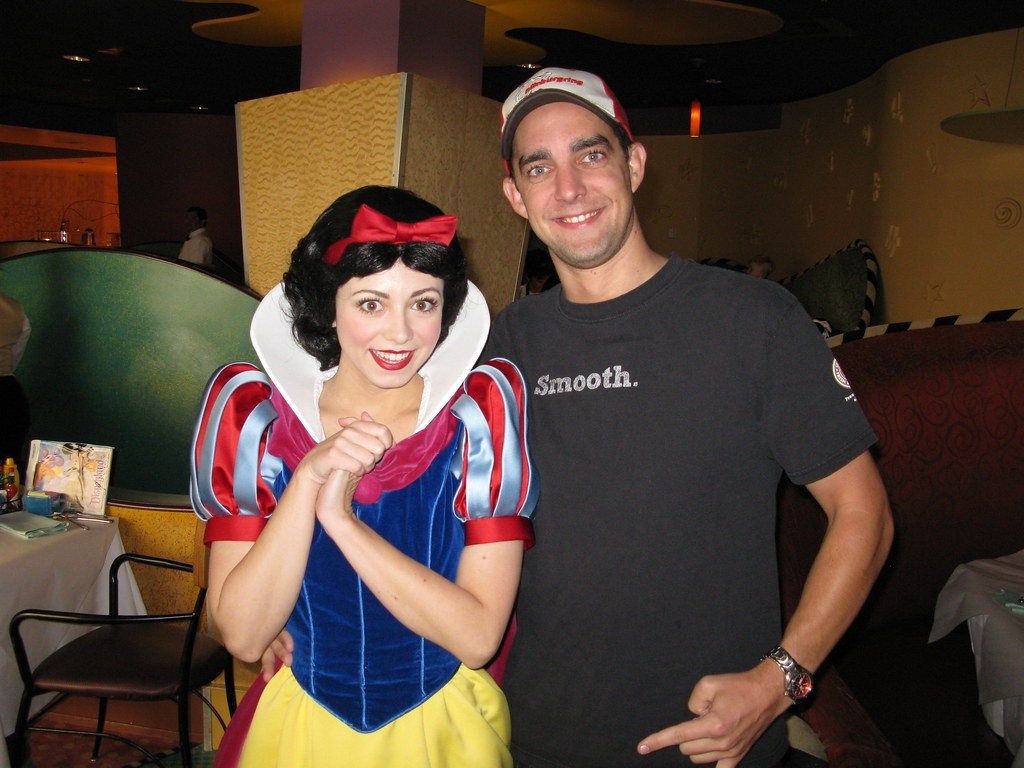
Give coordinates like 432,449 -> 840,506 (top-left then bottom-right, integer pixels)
498,67 -> 635,181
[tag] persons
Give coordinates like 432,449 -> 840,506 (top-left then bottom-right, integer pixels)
747,255 -> 772,278
190,183 -> 541,767
518,250 -> 560,299
180,205 -> 214,264
265,64 -> 898,767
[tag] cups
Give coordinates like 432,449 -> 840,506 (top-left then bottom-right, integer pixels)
82,231 -> 95,246
105,233 -> 120,247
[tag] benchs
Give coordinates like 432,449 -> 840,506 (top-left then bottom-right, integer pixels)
0,238 -> 264,629
699,238 -> 879,333
779,308 -> 1024,767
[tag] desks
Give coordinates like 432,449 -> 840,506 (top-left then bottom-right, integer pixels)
927,548 -> 1023,768
0,510 -> 147,739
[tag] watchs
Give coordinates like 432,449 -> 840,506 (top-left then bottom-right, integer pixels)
761,645 -> 814,704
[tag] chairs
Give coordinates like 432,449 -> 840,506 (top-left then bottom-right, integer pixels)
9,517 -> 237,768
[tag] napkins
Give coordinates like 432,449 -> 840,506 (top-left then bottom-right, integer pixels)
0,510 -> 68,540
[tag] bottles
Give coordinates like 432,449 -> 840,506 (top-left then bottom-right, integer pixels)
0,458 -> 19,510
60,222 -> 68,243
